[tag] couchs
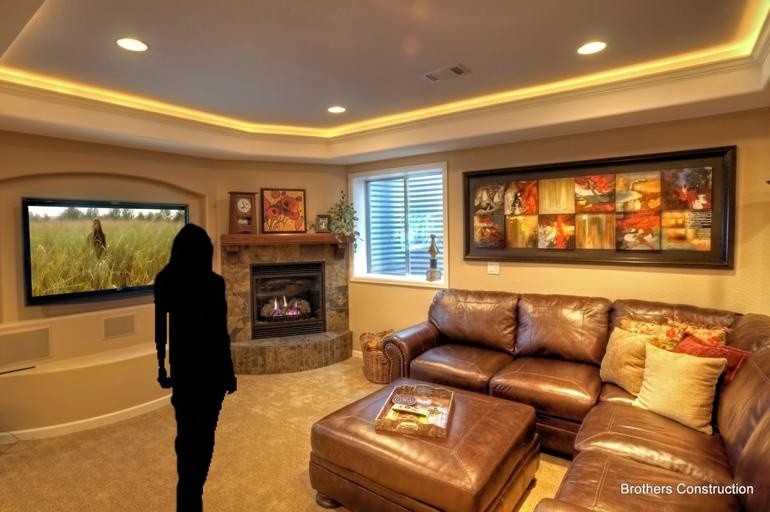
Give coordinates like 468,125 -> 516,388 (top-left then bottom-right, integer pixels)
382,289 -> 770,512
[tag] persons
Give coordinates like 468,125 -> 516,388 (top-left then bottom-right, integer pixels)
152,223 -> 237,511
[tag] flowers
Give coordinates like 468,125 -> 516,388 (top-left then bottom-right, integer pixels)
327,190 -> 359,236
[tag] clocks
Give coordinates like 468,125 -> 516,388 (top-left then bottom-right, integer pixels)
228,191 -> 259,234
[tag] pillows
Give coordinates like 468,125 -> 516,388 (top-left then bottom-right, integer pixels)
600,326 -> 660,398
670,332 -> 753,384
665,317 -> 732,345
632,342 -> 727,435
621,319 -> 685,351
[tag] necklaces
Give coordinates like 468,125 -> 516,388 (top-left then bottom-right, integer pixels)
87,218 -> 106,257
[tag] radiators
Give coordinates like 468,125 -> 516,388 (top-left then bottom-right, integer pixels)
309,377 -> 540,512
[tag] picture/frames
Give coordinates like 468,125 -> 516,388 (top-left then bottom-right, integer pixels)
260,189 -> 305,233
463,144 -> 736,270
315,214 -> 331,233
